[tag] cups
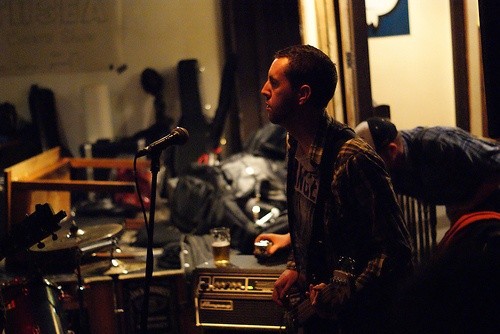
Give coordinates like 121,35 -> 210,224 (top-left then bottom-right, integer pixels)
253,240 -> 272,266
210,227 -> 231,267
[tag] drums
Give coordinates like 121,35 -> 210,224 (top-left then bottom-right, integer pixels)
75,259 -> 160,275
0,274 -> 88,334
82,269 -> 194,334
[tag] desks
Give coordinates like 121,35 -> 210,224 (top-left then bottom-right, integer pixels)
4,146 -> 151,235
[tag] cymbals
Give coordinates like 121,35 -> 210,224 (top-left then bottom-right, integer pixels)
91,246 -> 163,257
29,223 -> 123,252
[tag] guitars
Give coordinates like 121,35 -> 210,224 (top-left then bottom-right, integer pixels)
280,256 -> 356,334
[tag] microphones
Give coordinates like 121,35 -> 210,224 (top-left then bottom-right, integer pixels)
135,126 -> 190,158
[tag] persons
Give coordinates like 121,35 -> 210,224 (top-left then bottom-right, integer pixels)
254,116 -> 500,263
253,43 -> 415,334
